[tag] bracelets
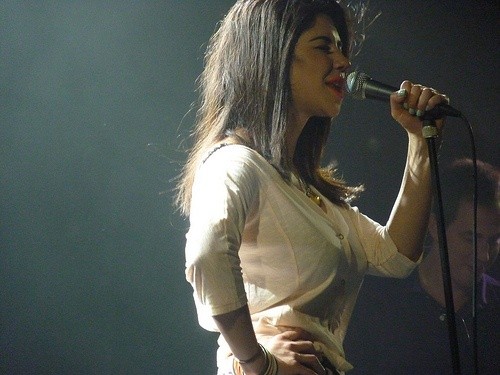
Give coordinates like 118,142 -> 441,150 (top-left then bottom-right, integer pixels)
233,349 -> 261,364
232,344 -> 278,375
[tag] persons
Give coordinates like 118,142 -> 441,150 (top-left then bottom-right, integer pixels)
343,156 -> 500,375
177,0 -> 450,374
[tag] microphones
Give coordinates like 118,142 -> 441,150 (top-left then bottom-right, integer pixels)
344,71 -> 463,119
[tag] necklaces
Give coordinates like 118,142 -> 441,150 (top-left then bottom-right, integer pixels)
300,179 -> 320,206
